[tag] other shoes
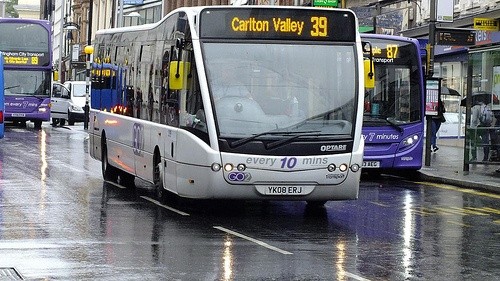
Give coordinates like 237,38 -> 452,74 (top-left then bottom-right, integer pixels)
482,156 -> 489,161
434,147 -> 439,152
470,157 -> 477,161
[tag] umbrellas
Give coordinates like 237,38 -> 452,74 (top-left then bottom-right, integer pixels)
441,86 -> 461,103
460,89 -> 499,106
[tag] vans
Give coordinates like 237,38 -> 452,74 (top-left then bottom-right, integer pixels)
50,81 -> 90,124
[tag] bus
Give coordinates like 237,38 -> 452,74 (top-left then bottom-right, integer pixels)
87,6 -> 365,206
359,33 -> 424,170
0,17 -> 51,130
0,51 -> 5,139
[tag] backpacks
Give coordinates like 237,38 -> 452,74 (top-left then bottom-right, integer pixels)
474,103 -> 493,125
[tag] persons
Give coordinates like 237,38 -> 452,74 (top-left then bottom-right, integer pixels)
426,99 -> 446,153
50,86 -> 65,127
469,94 -> 490,161
